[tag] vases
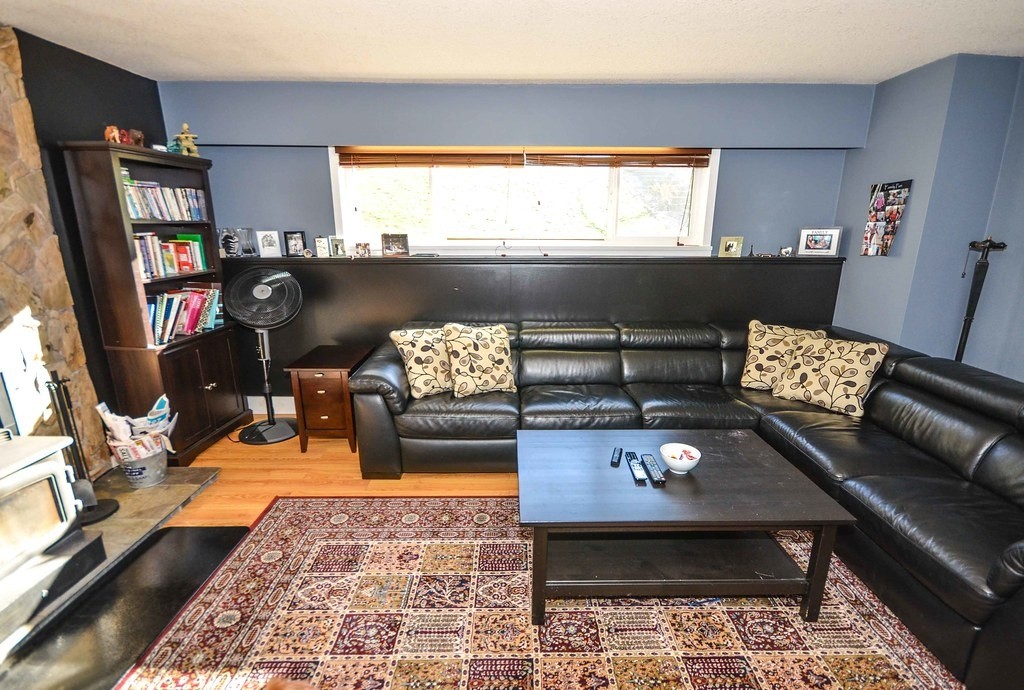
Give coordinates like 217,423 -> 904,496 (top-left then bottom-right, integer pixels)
233,228 -> 257,257
216,228 -> 240,256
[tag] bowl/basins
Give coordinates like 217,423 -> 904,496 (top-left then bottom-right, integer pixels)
660,442 -> 701,474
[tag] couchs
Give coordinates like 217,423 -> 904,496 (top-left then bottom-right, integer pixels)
350,323 -> 1024,690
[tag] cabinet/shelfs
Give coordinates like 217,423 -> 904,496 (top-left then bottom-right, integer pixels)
59,140 -> 255,467
284,345 -> 377,453
0,466 -> 250,690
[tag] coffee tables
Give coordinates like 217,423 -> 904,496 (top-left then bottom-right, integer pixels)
516,430 -> 857,625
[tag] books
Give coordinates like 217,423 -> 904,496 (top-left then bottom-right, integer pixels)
146,282 -> 223,345
134,232 -> 207,280
120,167 -> 209,221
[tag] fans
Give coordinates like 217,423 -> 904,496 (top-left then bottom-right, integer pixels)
223,266 -> 304,444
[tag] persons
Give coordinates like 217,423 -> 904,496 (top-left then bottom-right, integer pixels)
358,244 -> 370,257
318,241 -> 343,256
290,237 -> 300,253
807,235 -> 830,248
387,241 -> 405,251
263,235 -> 277,247
861,189 -> 908,256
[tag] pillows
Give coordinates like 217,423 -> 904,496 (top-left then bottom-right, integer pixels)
389,329 -> 453,399
740,320 -> 829,390
443,323 -> 517,398
775,335 -> 890,417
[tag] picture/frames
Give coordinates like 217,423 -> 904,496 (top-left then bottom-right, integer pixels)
718,236 -> 745,257
284,230 -> 307,257
797,225 -> 844,259
255,230 -> 282,258
328,235 -> 347,257
354,242 -> 373,258
382,233 -> 410,257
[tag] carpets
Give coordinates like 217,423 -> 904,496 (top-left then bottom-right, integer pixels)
114,495 -> 967,690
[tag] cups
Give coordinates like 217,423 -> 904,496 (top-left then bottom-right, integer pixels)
237,228 -> 257,256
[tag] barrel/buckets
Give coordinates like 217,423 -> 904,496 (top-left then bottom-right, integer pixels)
107,438 -> 170,488
